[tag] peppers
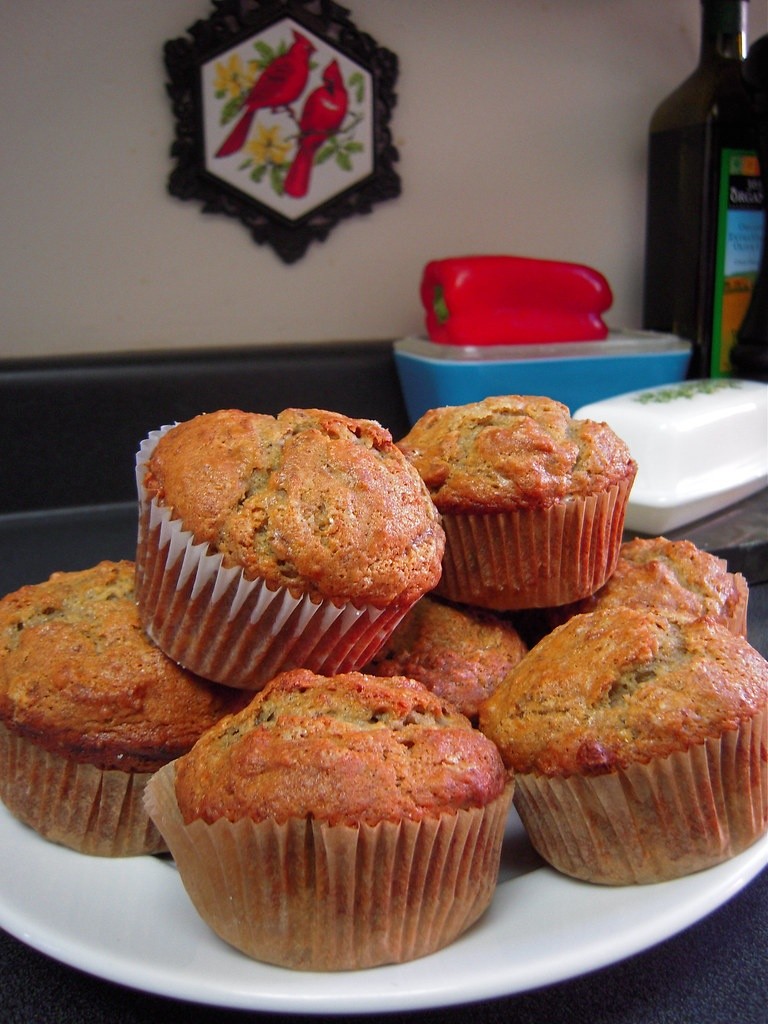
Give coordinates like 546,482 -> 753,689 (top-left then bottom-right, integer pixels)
419,255 -> 614,346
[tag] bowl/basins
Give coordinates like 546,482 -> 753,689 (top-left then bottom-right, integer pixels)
574,377 -> 767,536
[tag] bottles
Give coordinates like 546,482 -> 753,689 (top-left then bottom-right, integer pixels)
643,0 -> 768,380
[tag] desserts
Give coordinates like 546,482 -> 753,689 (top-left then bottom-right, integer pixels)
0,392 -> 767,971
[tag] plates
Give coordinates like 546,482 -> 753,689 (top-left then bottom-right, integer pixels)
0,801 -> 768,1016
